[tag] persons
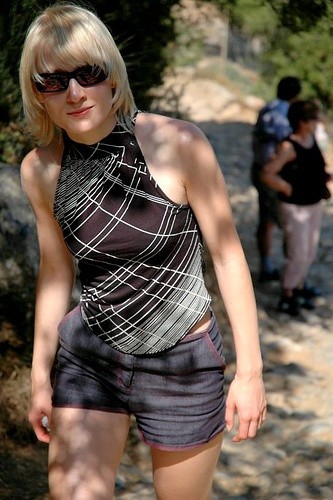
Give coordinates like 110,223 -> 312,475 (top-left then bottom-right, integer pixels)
250,76 -> 331,317
20,4 -> 269,500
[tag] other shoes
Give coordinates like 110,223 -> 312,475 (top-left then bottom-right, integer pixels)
277,286 -> 325,321
257,275 -> 281,291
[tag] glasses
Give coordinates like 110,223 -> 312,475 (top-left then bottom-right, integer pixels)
35,64 -> 108,93
